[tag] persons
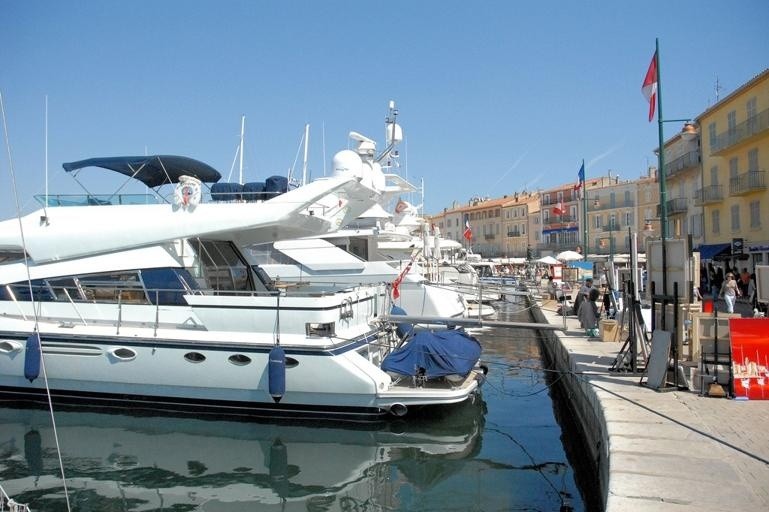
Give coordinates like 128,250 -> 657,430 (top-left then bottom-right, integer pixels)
577,289 -> 601,329
692,262 -> 757,308
489,261 -> 553,280
578,277 -> 598,294
717,272 -> 743,313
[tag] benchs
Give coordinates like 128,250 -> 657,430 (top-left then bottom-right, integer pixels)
641,51 -> 659,123
574,163 -> 586,200
463,219 -> 473,241
552,195 -> 568,216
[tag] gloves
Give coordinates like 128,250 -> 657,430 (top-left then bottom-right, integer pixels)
0,391 -> 491,512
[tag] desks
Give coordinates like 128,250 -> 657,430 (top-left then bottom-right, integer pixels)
582,198 -> 601,261
597,236 -> 616,263
656,114 -> 700,306
643,215 -> 671,241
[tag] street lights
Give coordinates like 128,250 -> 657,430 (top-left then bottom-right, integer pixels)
597,236 -> 616,263
582,198 -> 601,261
656,114 -> 700,306
643,215 -> 671,241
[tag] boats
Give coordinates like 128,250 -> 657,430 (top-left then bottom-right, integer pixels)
1,90 -> 508,336
0,99 -> 490,413
0,391 -> 491,512
